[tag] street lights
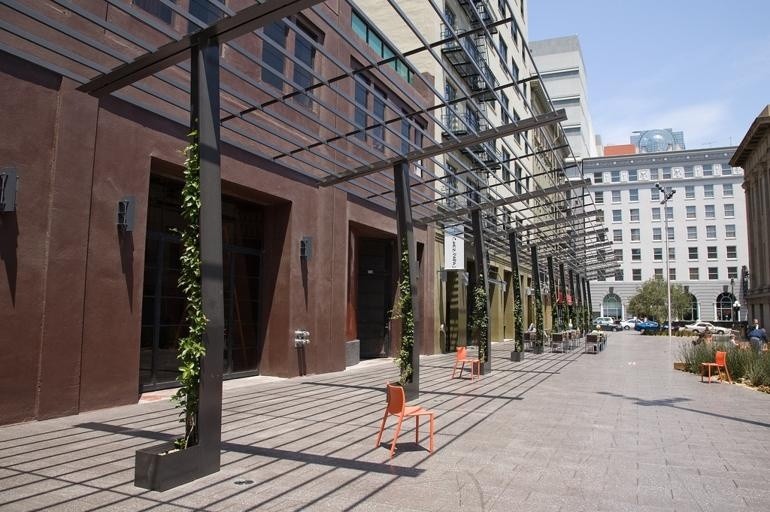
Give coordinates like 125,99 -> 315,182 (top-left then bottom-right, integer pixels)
731,278 -> 736,321
655,181 -> 677,336
733,300 -> 741,322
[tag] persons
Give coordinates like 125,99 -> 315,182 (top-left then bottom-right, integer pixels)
748,324 -> 769,343
590,324 -> 603,338
752,318 -> 763,330
527,319 -> 536,332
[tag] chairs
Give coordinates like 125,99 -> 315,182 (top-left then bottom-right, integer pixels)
523,329 -> 606,354
452,345 -> 481,382
700,349 -> 732,385
375,379 -> 436,457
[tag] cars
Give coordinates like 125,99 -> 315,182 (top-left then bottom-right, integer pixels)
592,315 -> 730,336
